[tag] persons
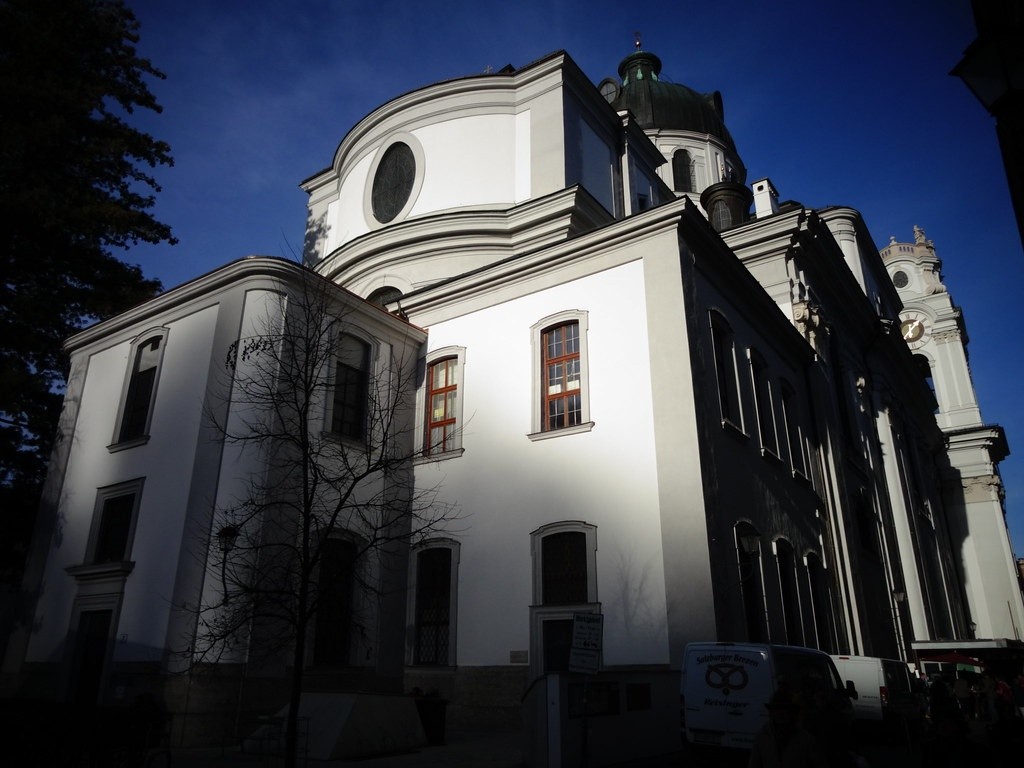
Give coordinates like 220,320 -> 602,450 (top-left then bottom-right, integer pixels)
919,671 -> 1024,768
748,668 -> 858,768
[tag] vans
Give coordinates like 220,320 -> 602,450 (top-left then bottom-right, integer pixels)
829,654 -> 923,739
679,640 -> 858,750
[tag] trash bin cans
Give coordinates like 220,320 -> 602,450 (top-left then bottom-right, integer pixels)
416,696 -> 447,746
